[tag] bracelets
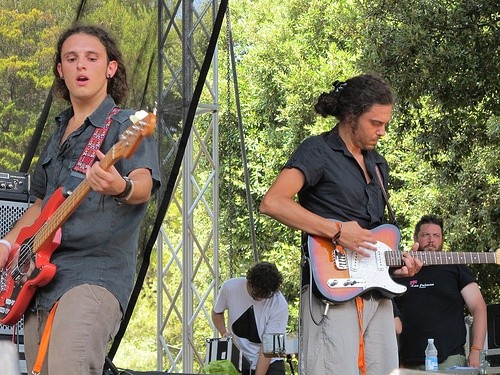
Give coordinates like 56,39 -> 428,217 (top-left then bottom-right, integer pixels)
332,222 -> 342,256
470,346 -> 484,351
1,239 -> 12,250
110,176 -> 133,201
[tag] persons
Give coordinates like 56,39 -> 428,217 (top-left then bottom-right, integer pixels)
259,75 -> 423,375
0,25 -> 161,375
212,262 -> 287,375
393,214 -> 487,368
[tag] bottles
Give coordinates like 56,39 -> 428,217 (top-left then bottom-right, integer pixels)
424,338 -> 439,372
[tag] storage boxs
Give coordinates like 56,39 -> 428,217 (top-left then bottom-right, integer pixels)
204,336 -> 253,375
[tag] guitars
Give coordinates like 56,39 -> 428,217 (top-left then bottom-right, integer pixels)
0,109 -> 156,327
301,217 -> 500,306
